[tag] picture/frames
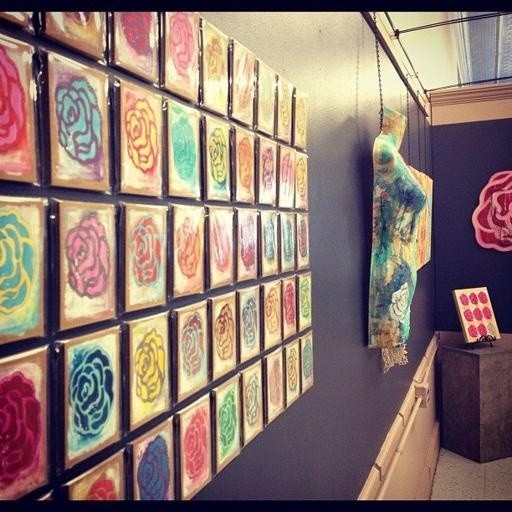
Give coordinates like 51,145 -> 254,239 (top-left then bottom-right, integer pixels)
1,196 -> 48,345
111,11 -> 159,86
0,33 -> 40,186
40,49 -> 111,196
57,447 -> 125,501
49,323 -> 125,473
126,415 -> 176,503
126,310 -> 174,435
42,12 -> 108,67
0,342 -> 51,501
49,196 -> 119,334
112,75 -> 164,200
119,200 -> 170,314
2,11 -> 41,41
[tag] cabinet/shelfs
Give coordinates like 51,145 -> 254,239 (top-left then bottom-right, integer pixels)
438,340 -> 512,464
